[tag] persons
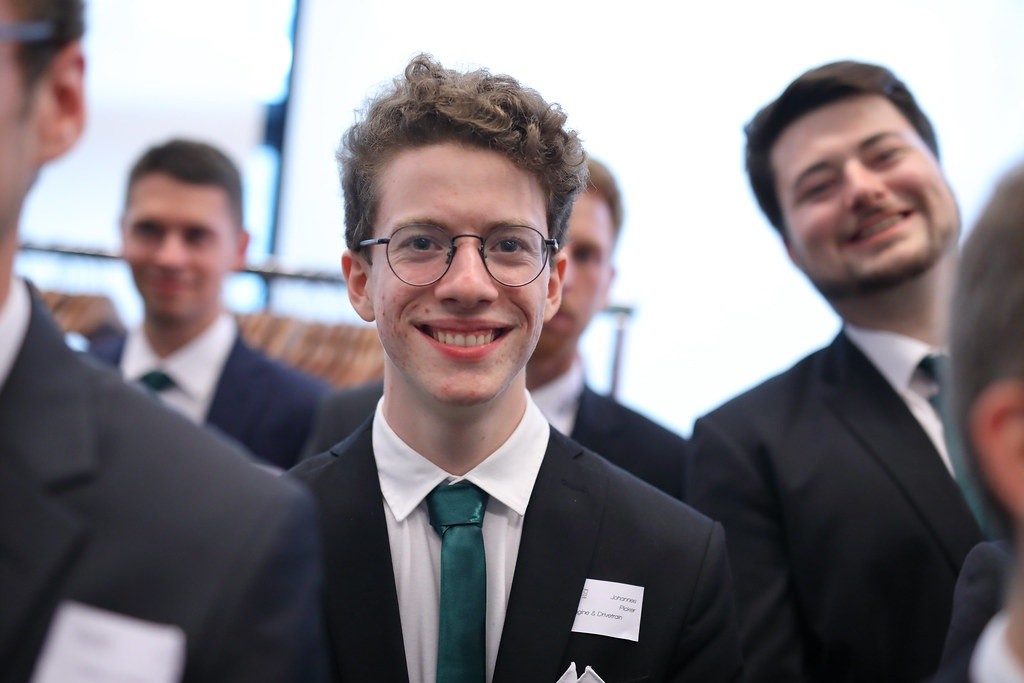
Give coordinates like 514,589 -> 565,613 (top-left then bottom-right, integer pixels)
936,158 -> 1024,683
0,1 -> 327,682
304,156 -> 686,501
82,139 -> 320,473
681,62 -> 989,683
286,52 -> 743,683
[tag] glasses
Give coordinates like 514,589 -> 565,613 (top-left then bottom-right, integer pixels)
356,224 -> 558,288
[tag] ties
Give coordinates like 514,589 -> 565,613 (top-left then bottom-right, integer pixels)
426,482 -> 489,683
919,355 -> 991,536
139,371 -> 173,394
